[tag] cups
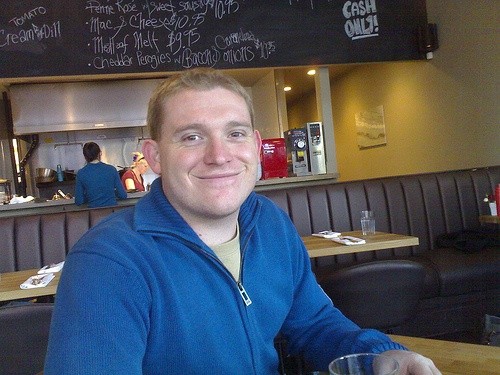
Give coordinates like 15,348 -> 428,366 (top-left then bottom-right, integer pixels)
360,210 -> 376,235
328,352 -> 399,375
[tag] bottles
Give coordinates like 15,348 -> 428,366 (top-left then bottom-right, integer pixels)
495,183 -> 500,215
57,164 -> 64,182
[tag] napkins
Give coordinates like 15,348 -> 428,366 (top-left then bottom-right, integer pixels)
330,236 -> 366,246
37,260 -> 64,274
311,230 -> 342,239
20,273 -> 55,289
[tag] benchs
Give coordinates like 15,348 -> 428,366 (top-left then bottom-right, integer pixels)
0,165 -> 500,346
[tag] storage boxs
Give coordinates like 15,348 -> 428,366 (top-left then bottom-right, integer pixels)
283,122 -> 326,177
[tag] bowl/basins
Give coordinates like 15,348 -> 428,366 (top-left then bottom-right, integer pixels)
36,168 -> 56,177
63,170 -> 74,181
34,176 -> 56,183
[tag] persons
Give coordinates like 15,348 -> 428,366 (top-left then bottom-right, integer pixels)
44,67 -> 443,375
75,142 -> 128,208
121,151 -> 149,192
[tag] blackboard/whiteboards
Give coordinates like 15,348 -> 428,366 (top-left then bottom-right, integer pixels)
0,0 -> 428,85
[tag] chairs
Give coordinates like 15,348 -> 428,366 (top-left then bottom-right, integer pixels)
0,301 -> 53,375
325,258 -> 425,335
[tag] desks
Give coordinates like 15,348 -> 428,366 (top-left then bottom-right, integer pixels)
384,334 -> 500,375
0,229 -> 419,302
